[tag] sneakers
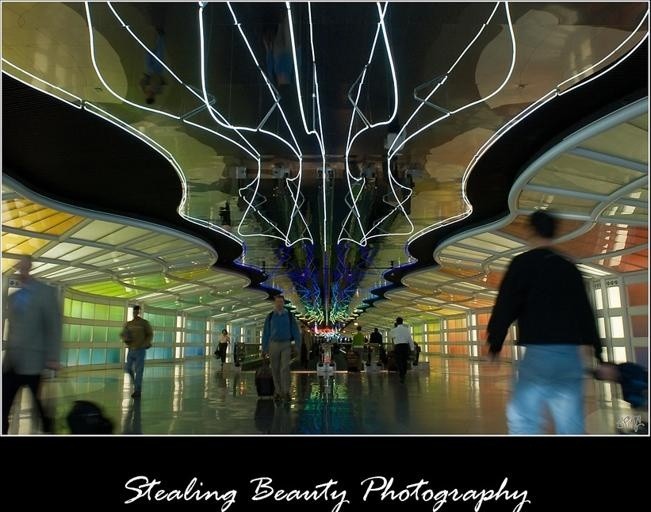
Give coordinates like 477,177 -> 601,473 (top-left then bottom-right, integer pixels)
131,391 -> 141,397
275,395 -> 292,402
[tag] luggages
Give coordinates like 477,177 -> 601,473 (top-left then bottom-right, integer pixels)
254,355 -> 275,398
54,365 -> 113,434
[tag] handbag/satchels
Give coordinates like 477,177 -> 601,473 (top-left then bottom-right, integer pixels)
215,346 -> 220,359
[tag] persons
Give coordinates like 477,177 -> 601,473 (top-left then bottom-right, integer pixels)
591,360 -> 648,410
389,317 -> 415,384
218,329 -> 230,365
2,254 -> 64,435
299,325 -> 313,369
120,305 -> 152,397
261,295 -> 302,400
330,326 -> 382,359
482,209 -> 603,434
